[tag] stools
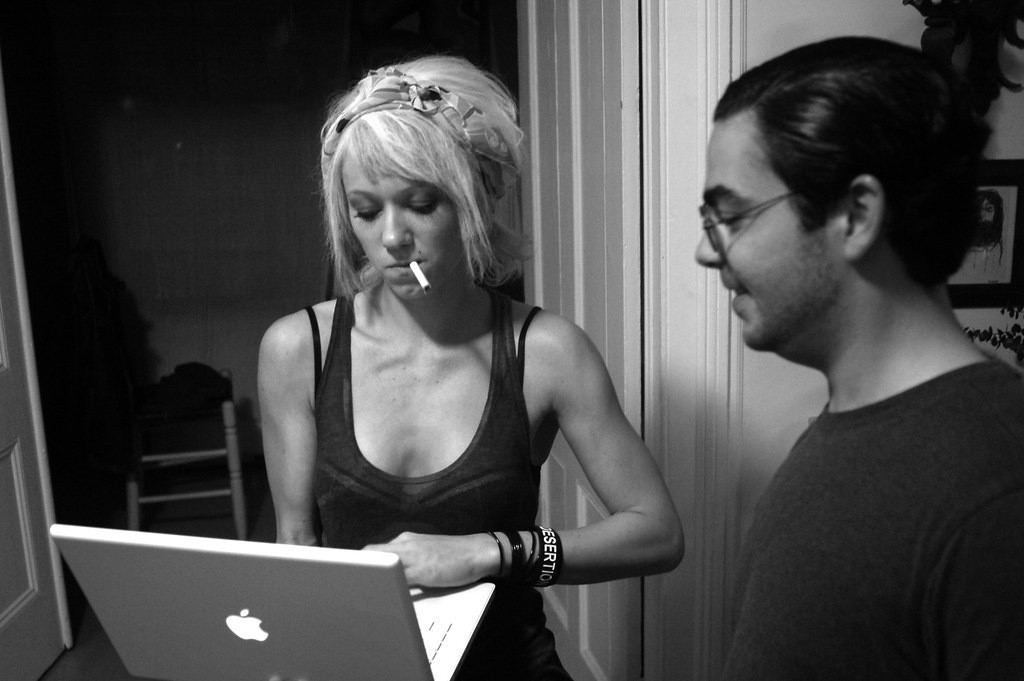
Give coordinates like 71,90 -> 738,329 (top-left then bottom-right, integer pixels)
123,372 -> 247,541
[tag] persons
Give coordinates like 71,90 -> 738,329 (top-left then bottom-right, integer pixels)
258,56 -> 686,681
696,37 -> 1024,680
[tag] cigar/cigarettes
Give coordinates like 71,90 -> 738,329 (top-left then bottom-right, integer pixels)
409,261 -> 431,293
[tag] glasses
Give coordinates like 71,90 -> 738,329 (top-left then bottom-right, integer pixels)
699,191 -> 820,251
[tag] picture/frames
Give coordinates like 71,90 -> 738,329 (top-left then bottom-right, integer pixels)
943,157 -> 1024,310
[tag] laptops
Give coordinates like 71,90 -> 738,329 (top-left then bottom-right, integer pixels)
47,523 -> 498,681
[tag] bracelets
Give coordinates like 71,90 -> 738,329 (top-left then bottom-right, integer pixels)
533,525 -> 560,586
488,529 -> 536,573
